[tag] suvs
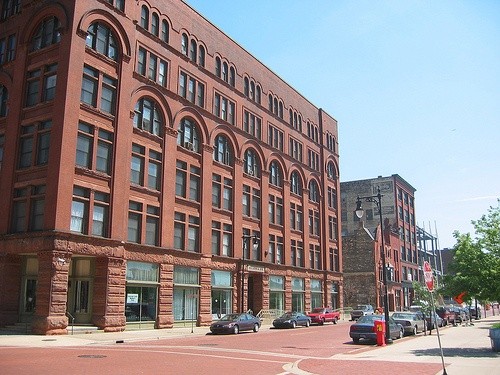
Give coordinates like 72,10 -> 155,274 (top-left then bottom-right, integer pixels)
351,304 -> 374,320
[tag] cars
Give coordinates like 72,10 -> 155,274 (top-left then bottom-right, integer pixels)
210,313 -> 262,334
391,305 -> 481,336
348,315 -> 403,346
273,311 -> 313,329
307,308 -> 340,325
489,328 -> 500,353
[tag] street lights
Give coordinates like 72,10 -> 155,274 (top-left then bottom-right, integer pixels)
241,234 -> 262,313
352,186 -> 393,344
